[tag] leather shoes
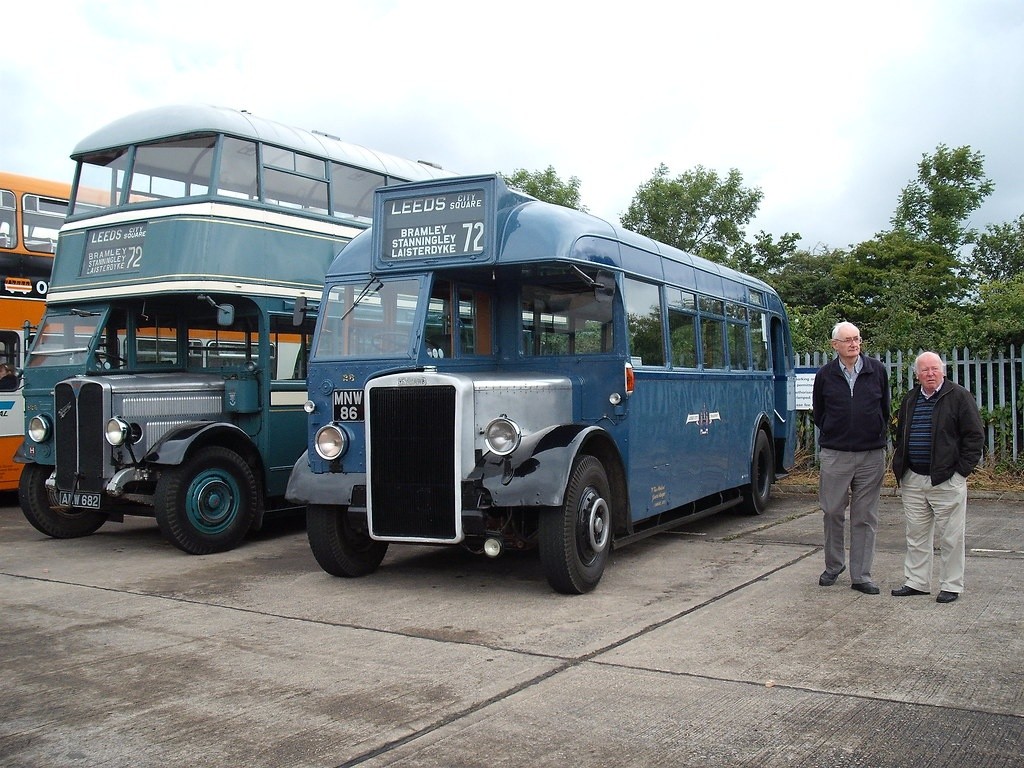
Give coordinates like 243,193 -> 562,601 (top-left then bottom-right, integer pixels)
891,586 -> 930,596
819,565 -> 846,586
936,590 -> 958,603
851,582 -> 880,594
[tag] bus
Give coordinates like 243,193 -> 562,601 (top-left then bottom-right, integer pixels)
13,99 -> 568,556
286,173 -> 797,596
0,172 -> 159,492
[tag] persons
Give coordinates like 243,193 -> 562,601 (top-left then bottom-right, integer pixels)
892,352 -> 984,603
0,363 -> 14,376
813,322 -> 890,594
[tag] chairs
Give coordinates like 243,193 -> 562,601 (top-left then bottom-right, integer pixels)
0,232 -> 54,253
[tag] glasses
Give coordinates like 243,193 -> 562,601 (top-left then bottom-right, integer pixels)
835,337 -> 862,345
